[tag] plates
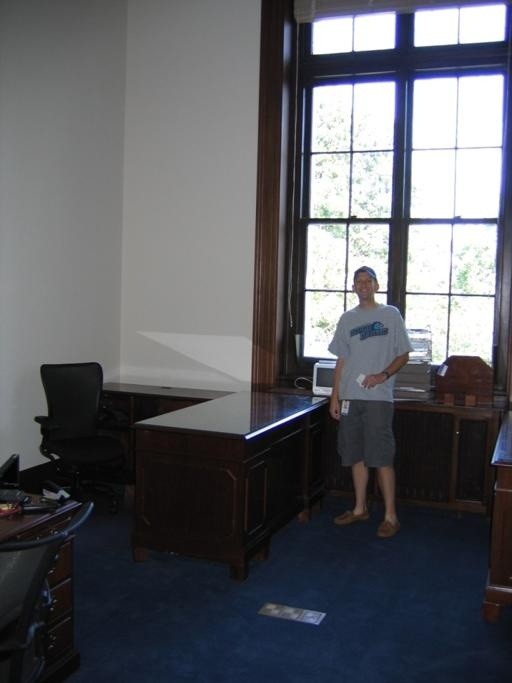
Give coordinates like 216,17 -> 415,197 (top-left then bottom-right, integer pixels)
322,399 -> 505,514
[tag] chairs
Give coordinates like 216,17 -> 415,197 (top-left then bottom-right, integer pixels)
334,510 -> 369,525
377,520 -> 401,538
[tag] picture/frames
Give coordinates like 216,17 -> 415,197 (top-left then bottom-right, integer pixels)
0,499 -> 94,683
99,382 -> 330,581
479,410 -> 512,624
1,494 -> 82,682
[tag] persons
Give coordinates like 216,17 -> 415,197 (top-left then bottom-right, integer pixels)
327,266 -> 414,539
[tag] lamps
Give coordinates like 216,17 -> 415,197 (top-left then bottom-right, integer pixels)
34,362 -> 136,514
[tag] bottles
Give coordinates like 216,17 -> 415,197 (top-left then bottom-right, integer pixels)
355,266 -> 376,280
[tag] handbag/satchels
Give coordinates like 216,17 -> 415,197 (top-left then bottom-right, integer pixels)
380,371 -> 390,380
